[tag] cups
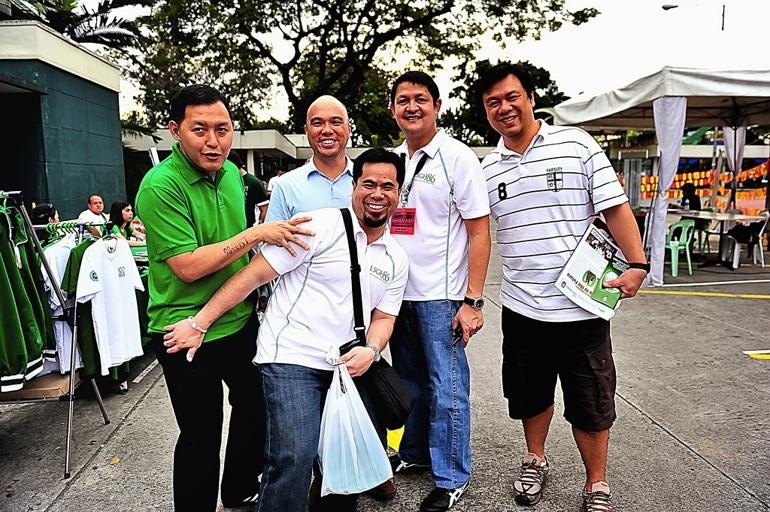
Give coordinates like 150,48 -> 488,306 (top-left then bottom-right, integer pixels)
711,196 -> 726,216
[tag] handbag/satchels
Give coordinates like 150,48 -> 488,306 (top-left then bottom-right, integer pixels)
340,339 -> 415,430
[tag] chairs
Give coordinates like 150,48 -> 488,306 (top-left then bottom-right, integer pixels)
720,211 -> 770,268
702,209 -> 743,255
666,218 -> 696,277
682,207 -> 714,249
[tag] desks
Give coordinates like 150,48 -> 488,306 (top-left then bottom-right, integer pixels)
677,211 -> 759,271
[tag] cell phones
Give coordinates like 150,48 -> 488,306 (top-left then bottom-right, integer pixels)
450,326 -> 463,347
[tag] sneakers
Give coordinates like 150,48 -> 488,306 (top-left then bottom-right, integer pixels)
369,481 -> 399,502
514,453 -> 549,505
385,454 -> 433,477
418,481 -> 470,511
582,481 -> 612,512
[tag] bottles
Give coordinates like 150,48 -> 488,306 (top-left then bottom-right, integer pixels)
684,198 -> 690,212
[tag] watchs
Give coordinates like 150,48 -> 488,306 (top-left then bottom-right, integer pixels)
188,314 -> 209,334
464,296 -> 485,311
365,344 -> 382,362
632,261 -> 650,273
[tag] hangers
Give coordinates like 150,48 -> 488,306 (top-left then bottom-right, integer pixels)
0,187 -> 117,246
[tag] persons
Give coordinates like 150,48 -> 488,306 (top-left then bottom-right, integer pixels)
671,183 -> 704,241
267,166 -> 287,196
109,202 -> 147,250
229,149 -> 267,226
479,61 -> 650,511
167,146 -> 414,512
376,70 -> 489,512
134,84 -> 319,512
29,202 -> 59,227
262,93 -> 404,506
78,194 -> 112,236
